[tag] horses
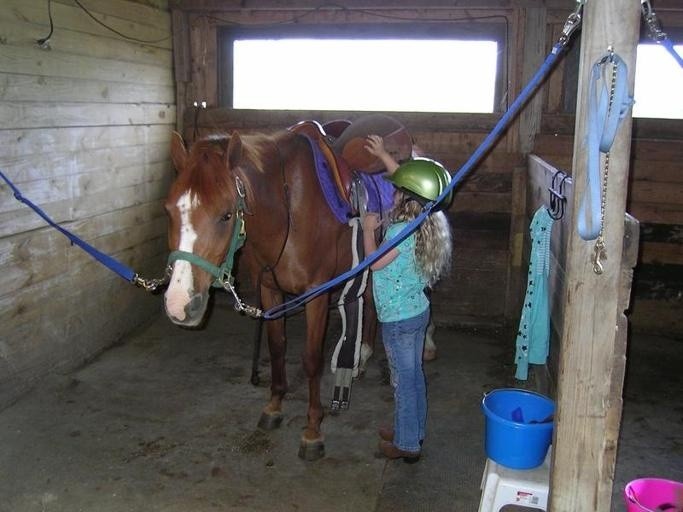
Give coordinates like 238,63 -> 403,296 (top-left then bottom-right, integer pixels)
163,121 -> 439,462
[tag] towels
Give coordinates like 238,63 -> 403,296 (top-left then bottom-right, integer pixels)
513,205 -> 554,382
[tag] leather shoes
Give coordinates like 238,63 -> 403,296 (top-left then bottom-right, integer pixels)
378,424 -> 423,464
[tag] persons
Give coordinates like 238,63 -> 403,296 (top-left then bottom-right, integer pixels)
362,134 -> 453,463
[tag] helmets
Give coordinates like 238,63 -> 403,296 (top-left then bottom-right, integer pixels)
382,158 -> 453,209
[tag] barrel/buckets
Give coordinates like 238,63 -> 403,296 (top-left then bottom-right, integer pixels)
623,477 -> 683,512
481,387 -> 555,470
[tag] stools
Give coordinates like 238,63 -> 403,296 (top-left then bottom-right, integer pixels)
478,444 -> 552,511
